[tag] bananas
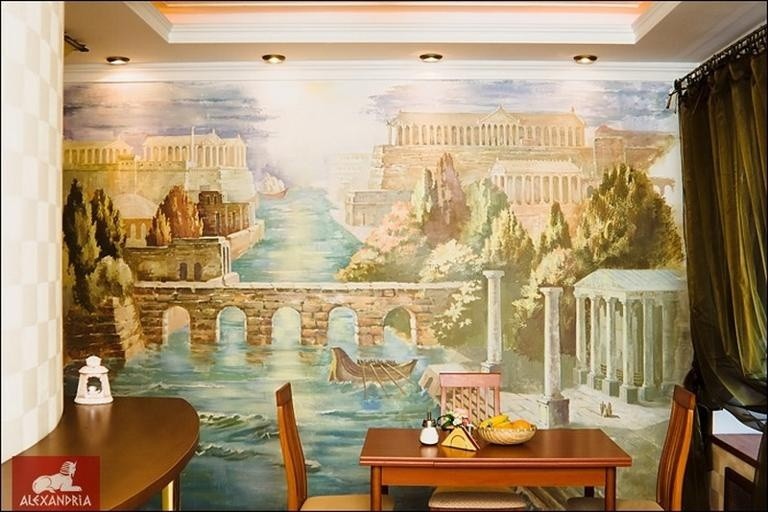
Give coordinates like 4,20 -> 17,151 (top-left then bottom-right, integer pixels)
480,416 -> 507,429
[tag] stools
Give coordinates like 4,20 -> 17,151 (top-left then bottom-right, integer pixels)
428,372 -> 531,512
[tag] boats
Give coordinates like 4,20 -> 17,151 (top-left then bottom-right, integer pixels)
327,347 -> 417,386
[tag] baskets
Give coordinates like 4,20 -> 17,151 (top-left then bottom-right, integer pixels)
477,425 -> 538,446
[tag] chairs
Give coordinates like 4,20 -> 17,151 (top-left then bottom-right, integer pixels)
275,381 -> 396,512
568,384 -> 696,511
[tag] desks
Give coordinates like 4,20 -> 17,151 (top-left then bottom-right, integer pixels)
2,394 -> 201,512
359,427 -> 632,512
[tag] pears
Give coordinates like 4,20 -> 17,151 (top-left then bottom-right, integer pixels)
510,420 -> 532,428
